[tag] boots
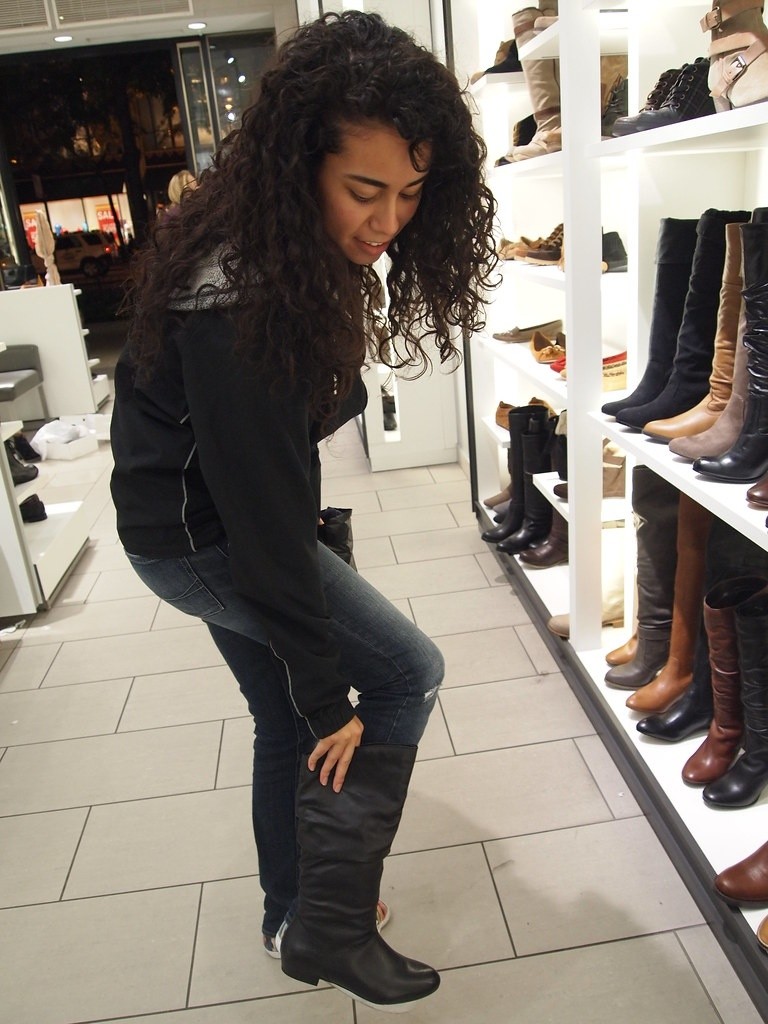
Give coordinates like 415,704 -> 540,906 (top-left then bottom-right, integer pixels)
482,405 -> 568,568
280,744 -> 441,1005
601,74 -> 628,139
546,207 -> 768,809
699,0 -> 768,113
505,0 -> 561,160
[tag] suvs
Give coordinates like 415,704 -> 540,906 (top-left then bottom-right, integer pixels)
0,231 -> 114,283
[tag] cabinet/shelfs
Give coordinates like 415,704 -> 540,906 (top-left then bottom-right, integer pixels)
0,283 -> 111,416
443,0 -> 768,1024
339,0 -> 460,471
0,419 -> 94,619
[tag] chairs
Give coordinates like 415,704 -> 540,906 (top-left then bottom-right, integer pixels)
0,344 -> 50,427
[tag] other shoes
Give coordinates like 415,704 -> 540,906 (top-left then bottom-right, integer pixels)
470,40 -> 522,82
495,401 -> 518,431
492,319 -> 562,343
530,331 -> 566,363
528,397 -> 557,417
264,901 -> 390,959
714,841 -> 768,952
13,434 -> 40,462
493,115 -> 537,166
552,439 -> 626,498
2,440 -> 38,486
560,360 -> 626,392
548,577 -> 624,636
550,350 -> 627,373
20,495 -> 47,524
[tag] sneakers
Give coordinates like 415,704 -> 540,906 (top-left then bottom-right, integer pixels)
497,224 -> 628,272
611,57 -> 710,136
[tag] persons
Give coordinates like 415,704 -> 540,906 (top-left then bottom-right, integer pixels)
166,170 -> 196,206
110,9 -> 504,1012
